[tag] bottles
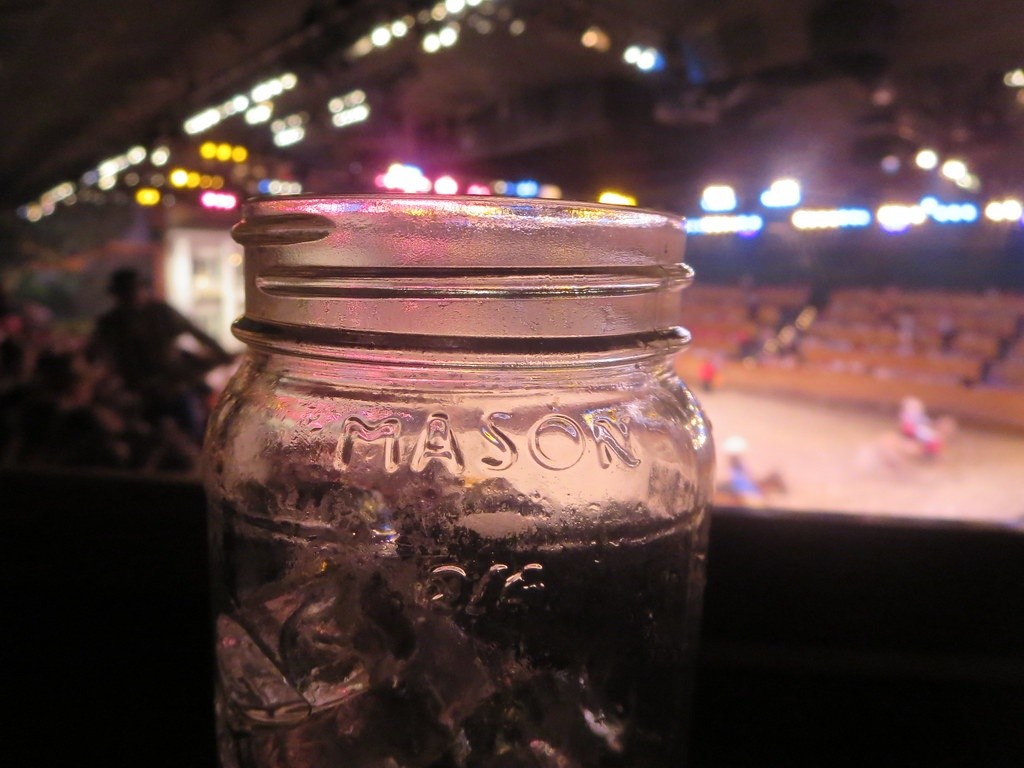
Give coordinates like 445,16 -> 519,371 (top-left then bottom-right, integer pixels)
198,196 -> 714,768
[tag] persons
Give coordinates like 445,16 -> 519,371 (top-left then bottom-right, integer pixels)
903,396 -> 938,457
700,356 -> 714,388
738,279 -> 958,365
0,261 -> 228,484
718,442 -> 762,507
